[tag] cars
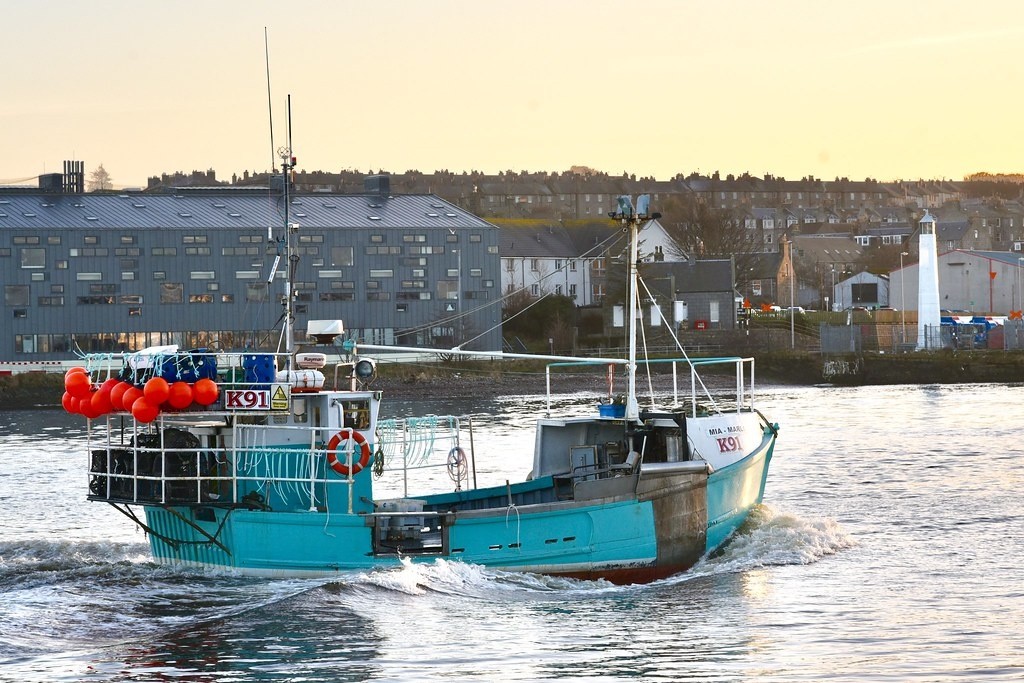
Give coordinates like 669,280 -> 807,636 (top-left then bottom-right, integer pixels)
736,305 -> 898,315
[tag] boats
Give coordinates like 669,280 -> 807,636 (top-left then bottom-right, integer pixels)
61,25 -> 781,589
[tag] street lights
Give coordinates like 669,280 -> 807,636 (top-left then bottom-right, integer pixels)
901,252 -> 908,342
787,240 -> 795,350
831,269 -> 835,304
451,250 -> 462,348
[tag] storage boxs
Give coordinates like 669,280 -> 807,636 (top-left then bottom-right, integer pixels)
378,500 -> 424,540
597,405 -> 625,418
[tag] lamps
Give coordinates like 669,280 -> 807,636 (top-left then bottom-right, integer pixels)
355,358 -> 377,391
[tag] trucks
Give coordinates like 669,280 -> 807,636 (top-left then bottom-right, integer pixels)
940,316 -> 1009,344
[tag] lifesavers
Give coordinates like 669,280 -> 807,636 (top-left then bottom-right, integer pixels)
327,430 -> 370,474
